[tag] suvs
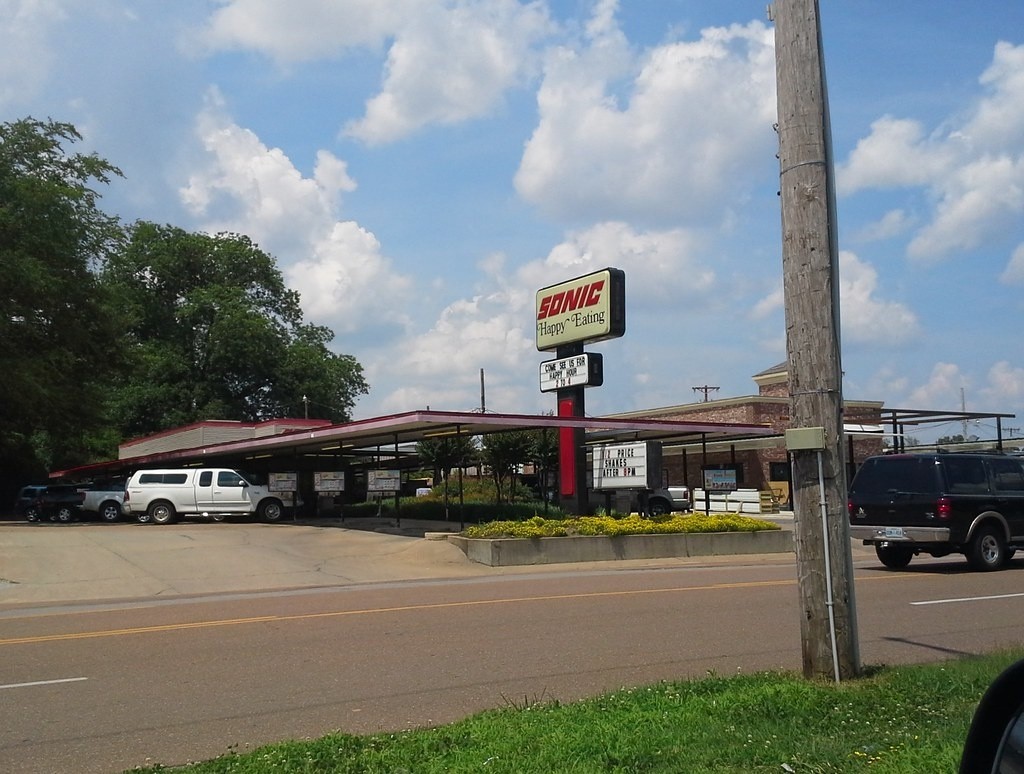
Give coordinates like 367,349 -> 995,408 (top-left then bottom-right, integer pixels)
848,448 -> 1024,572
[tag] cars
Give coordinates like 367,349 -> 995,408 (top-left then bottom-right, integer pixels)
545,473 -> 689,517
13,484 -> 104,523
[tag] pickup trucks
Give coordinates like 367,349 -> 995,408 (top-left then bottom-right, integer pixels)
121,468 -> 294,524
76,477 -> 151,523
